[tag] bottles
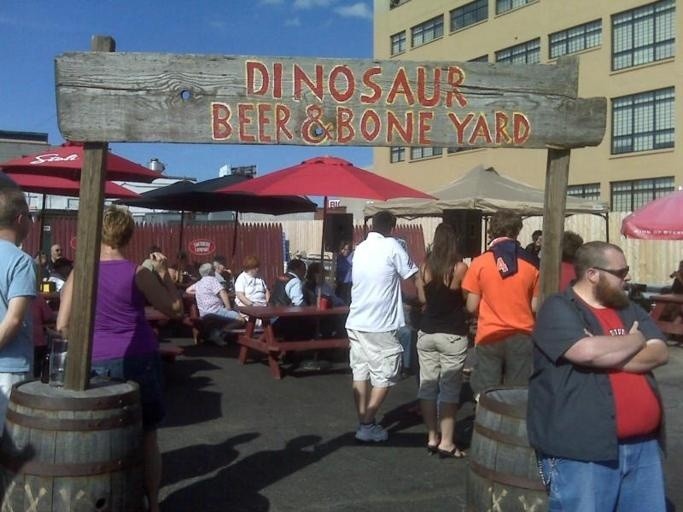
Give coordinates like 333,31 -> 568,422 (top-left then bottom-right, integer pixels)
39,348 -> 49,383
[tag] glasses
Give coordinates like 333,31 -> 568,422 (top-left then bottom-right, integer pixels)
590,266 -> 629,279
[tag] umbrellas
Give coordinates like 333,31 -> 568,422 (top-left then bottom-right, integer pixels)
209,151 -> 438,263
0,166 -> 144,248
149,170 -> 322,258
621,186 -> 683,241
114,173 -> 206,254
0,139 -> 163,188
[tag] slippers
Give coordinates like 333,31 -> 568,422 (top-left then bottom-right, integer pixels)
438,445 -> 468,459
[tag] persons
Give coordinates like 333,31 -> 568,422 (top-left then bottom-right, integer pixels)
528,228 -> 586,315
396,289 -> 419,380
47,243 -> 73,281
524,228 -> 542,258
525,239 -> 672,511
665,259 -> 682,347
0,170 -> 39,448
459,208 -> 540,400
415,221 -> 469,460
343,209 -> 419,445
33,290 -> 57,382
53,204 -> 183,510
32,250 -> 54,284
138,242 -> 354,373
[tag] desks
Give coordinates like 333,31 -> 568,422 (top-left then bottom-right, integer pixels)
648,294 -> 683,343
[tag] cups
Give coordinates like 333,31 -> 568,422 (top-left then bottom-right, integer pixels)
319,295 -> 331,310
463,256 -> 471,267
47,338 -> 69,387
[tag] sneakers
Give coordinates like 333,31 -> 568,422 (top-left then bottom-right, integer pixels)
354,423 -> 389,442
209,333 -> 228,346
426,444 -> 438,456
